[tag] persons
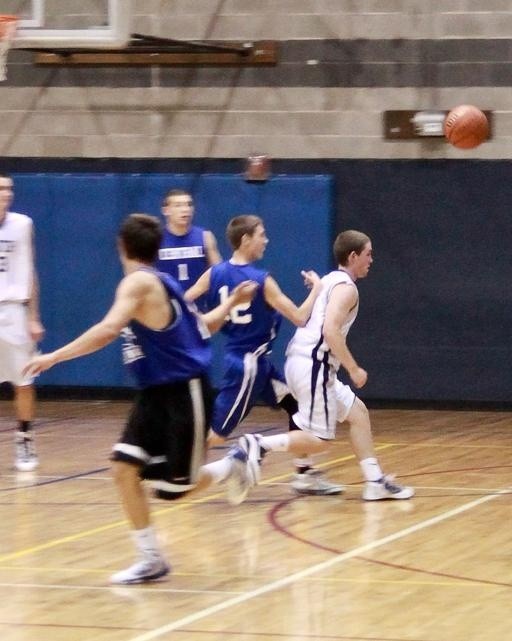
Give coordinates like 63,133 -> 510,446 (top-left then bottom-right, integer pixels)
237,230 -> 415,501
151,187 -> 226,312
183,213 -> 346,495
0,172 -> 47,471
19,212 -> 251,586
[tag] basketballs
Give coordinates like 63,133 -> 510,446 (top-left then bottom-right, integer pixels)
444,105 -> 488,148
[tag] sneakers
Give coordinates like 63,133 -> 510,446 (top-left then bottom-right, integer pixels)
363,482 -> 416,502
14,430 -> 39,473
110,562 -> 171,585
292,471 -> 346,496
225,445 -> 248,508
239,432 -> 265,488
